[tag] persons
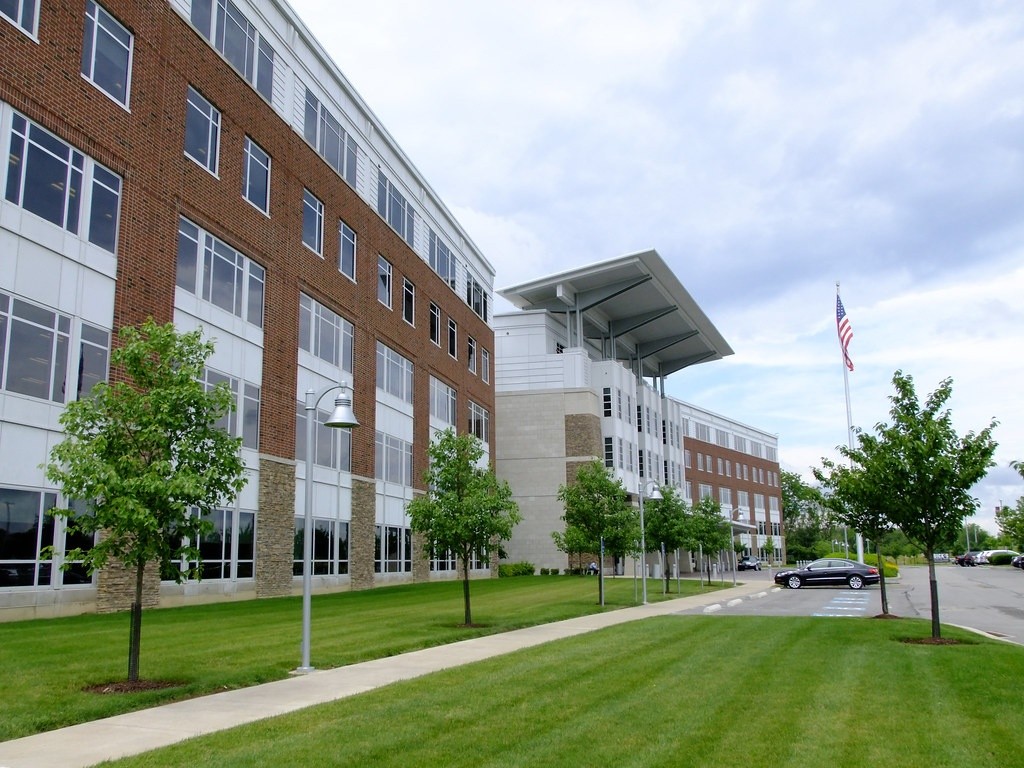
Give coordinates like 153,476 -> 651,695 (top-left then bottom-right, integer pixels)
588,561 -> 599,575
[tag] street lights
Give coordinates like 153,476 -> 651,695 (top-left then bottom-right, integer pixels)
729,507 -> 746,586
297,380 -> 361,675
638,480 -> 665,605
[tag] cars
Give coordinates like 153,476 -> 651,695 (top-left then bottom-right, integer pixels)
954,550 -> 1024,571
774,557 -> 880,590
737,555 -> 763,572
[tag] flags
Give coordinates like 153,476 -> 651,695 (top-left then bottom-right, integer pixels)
837,294 -> 854,371
